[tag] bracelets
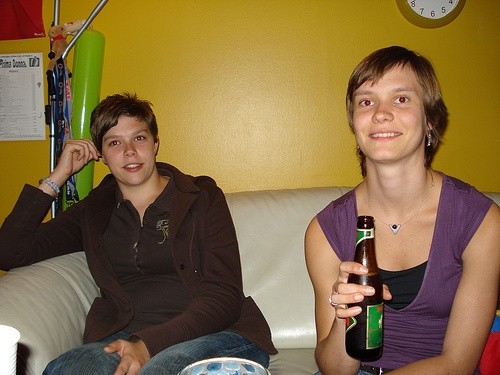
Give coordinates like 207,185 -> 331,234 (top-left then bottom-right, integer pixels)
43,177 -> 60,193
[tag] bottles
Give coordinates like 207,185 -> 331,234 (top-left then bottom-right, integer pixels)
345,215 -> 383,362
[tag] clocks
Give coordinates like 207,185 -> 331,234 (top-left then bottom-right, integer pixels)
395,0 -> 466,29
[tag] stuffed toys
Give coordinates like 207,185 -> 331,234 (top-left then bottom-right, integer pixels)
46,25 -> 72,78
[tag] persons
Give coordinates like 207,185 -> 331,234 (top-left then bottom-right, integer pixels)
0,92 -> 278,375
304,45 -> 500,375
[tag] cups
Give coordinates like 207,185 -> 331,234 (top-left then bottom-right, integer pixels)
0,324 -> 21,375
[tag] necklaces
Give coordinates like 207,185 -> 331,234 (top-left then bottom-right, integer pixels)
363,168 -> 434,234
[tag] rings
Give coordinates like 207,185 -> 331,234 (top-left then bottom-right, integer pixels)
328,294 -> 338,305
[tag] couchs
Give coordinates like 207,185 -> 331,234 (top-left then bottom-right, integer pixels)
0,186 -> 500,375
